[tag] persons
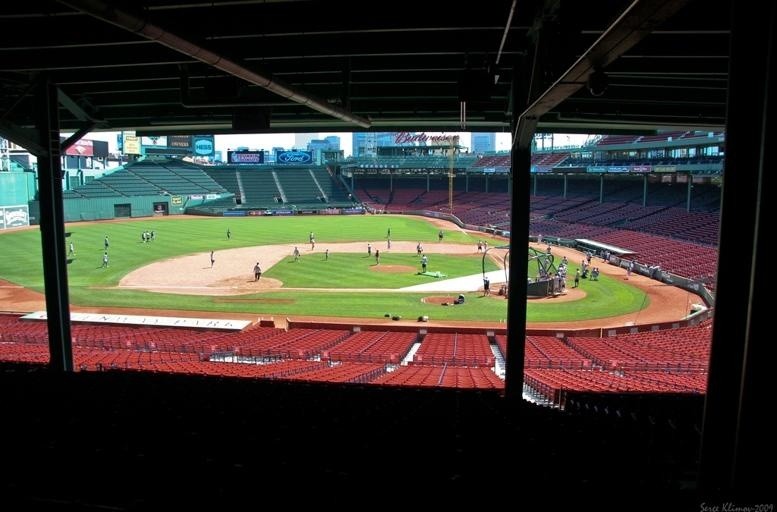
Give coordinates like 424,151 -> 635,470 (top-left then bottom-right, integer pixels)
538,234 -> 610,296
440,229 -> 444,240
310,232 -> 315,250
484,277 -> 490,297
628,262 -> 633,276
388,239 -> 392,248
478,241 -> 488,252
422,254 -> 428,273
210,250 -> 215,265
142,230 -> 156,241
102,252 -> 108,268
416,243 -> 424,254
325,250 -> 329,259
68,242 -> 73,254
367,244 -> 372,255
293,247 -> 300,260
105,236 -> 109,249
227,228 -> 232,239
387,227 -> 391,237
374,249 -> 381,264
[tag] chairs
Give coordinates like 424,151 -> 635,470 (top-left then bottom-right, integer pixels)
358,188 -> 673,243
353,149 -> 571,174
64,161 -> 355,206
587,134 -> 684,145
619,211 -> 718,301
2,310 -> 711,399
